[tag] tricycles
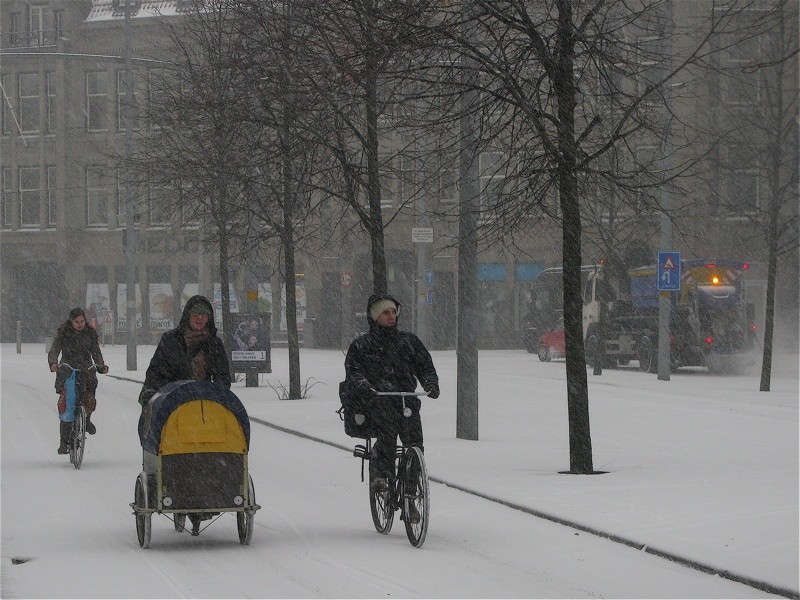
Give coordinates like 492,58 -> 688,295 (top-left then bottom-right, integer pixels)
129,379 -> 262,549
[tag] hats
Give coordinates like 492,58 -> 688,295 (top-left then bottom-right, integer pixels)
369,300 -> 397,322
189,301 -> 210,314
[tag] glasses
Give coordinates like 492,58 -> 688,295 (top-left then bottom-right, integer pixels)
189,310 -> 210,319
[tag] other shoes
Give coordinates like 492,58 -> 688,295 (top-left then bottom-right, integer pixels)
374,477 -> 388,490
398,499 -> 420,523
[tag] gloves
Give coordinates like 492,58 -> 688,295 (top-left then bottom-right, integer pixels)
424,384 -> 439,399
363,387 -> 377,400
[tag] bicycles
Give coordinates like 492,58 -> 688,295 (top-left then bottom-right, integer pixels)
51,362 -> 103,470
364,387 -> 432,548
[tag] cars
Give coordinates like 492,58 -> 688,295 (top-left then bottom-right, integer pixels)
536,316 -> 566,363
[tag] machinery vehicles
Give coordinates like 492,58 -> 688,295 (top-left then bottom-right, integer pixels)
582,258 -> 757,374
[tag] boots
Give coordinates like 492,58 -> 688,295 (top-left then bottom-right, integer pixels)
85,416 -> 96,435
58,439 -> 68,453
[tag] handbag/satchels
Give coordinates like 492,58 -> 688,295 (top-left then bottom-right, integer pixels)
339,382 -> 377,438
57,369 -> 76,422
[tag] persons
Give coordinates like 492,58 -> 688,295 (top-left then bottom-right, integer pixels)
232,318 -> 264,351
48,308 -> 108,454
138,295 -> 232,407
345,294 -> 439,524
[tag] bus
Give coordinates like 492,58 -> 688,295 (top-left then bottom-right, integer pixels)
523,264 -> 604,355
